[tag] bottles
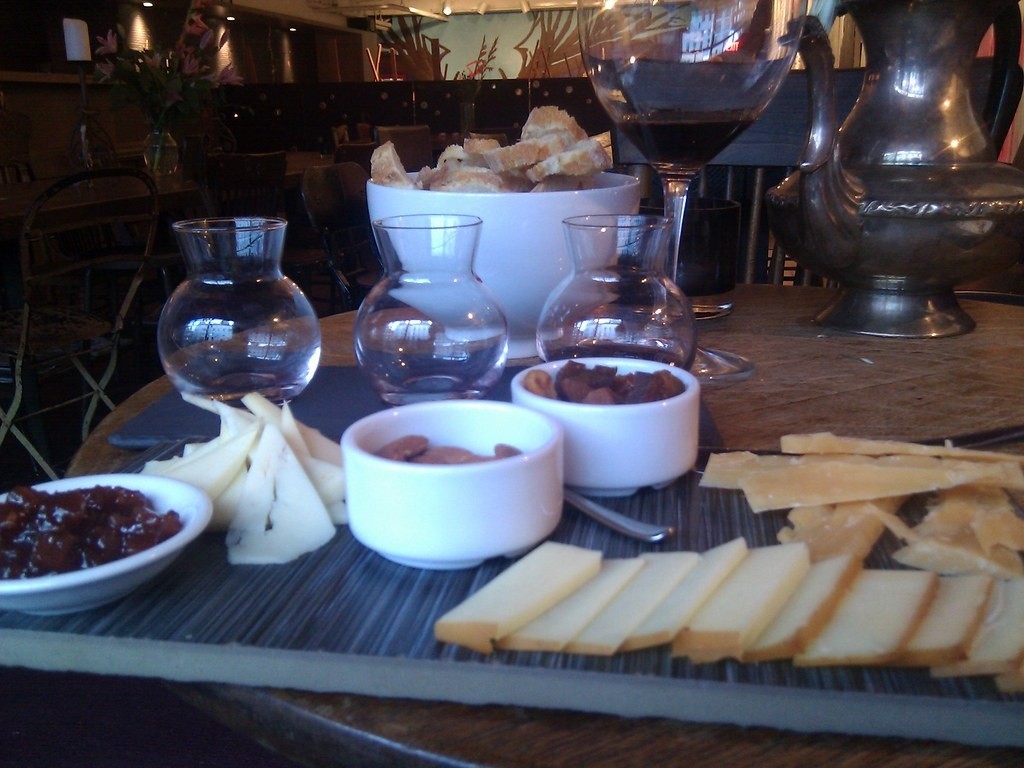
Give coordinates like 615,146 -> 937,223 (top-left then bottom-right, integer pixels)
536,212 -> 700,386
354,213 -> 509,405
155,216 -> 322,412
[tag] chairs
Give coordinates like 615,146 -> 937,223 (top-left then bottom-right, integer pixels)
0,114 -> 561,489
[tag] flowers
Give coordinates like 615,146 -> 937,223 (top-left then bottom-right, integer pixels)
90,2 -> 246,133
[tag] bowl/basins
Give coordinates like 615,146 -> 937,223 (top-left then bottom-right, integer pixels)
340,398 -> 566,569
510,356 -> 701,498
367,170 -> 641,362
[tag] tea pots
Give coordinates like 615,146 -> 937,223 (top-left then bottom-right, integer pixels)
778,0 -> 1024,336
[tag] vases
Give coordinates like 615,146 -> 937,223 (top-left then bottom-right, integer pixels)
141,111 -> 179,178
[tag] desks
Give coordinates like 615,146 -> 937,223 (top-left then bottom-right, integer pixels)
65,286 -> 1022,768
2,174 -> 219,225
281,139 -> 332,194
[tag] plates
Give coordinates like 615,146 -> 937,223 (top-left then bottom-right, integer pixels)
0,473 -> 214,613
108,363 -> 728,488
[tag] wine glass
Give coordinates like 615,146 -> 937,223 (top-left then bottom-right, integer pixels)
574,0 -> 809,389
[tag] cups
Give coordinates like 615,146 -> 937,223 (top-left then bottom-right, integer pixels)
641,192 -> 743,321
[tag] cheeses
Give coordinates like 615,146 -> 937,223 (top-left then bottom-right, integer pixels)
140,389 -> 347,563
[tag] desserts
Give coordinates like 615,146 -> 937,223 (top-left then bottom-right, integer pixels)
698,431 -> 1023,576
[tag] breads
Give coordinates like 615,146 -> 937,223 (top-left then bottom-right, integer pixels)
370,106 -> 610,190
434,538 -> 1024,692
